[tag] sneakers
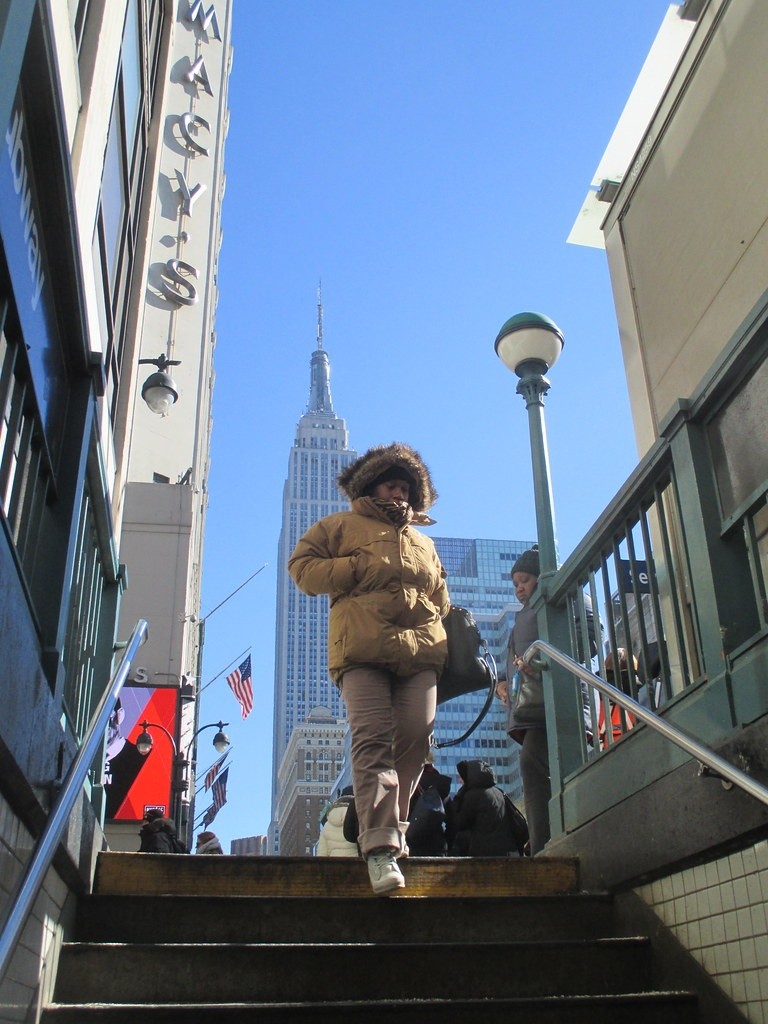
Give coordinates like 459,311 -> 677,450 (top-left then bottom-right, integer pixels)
367,847 -> 404,893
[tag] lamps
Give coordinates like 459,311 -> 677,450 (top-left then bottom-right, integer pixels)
138,353 -> 182,413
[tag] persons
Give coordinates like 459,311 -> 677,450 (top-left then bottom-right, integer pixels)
593,648 -> 639,750
493,543 -> 604,859
195,830 -> 223,855
137,808 -> 178,853
315,749 -> 531,858
288,440 -> 452,896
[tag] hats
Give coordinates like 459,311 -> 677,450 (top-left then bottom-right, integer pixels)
367,467 -> 413,486
510,544 -> 540,576
594,648 -> 638,675
143,809 -> 163,823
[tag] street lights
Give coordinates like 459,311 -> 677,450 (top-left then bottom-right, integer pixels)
493,311 -> 564,581
134,717 -> 231,852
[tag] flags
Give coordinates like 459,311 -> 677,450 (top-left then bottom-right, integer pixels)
202,751 -> 230,791
226,654 -> 255,720
203,767 -> 228,830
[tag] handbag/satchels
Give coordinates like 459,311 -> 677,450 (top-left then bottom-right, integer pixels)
496,786 -> 529,846
406,787 -> 445,848
431,606 -> 497,706
